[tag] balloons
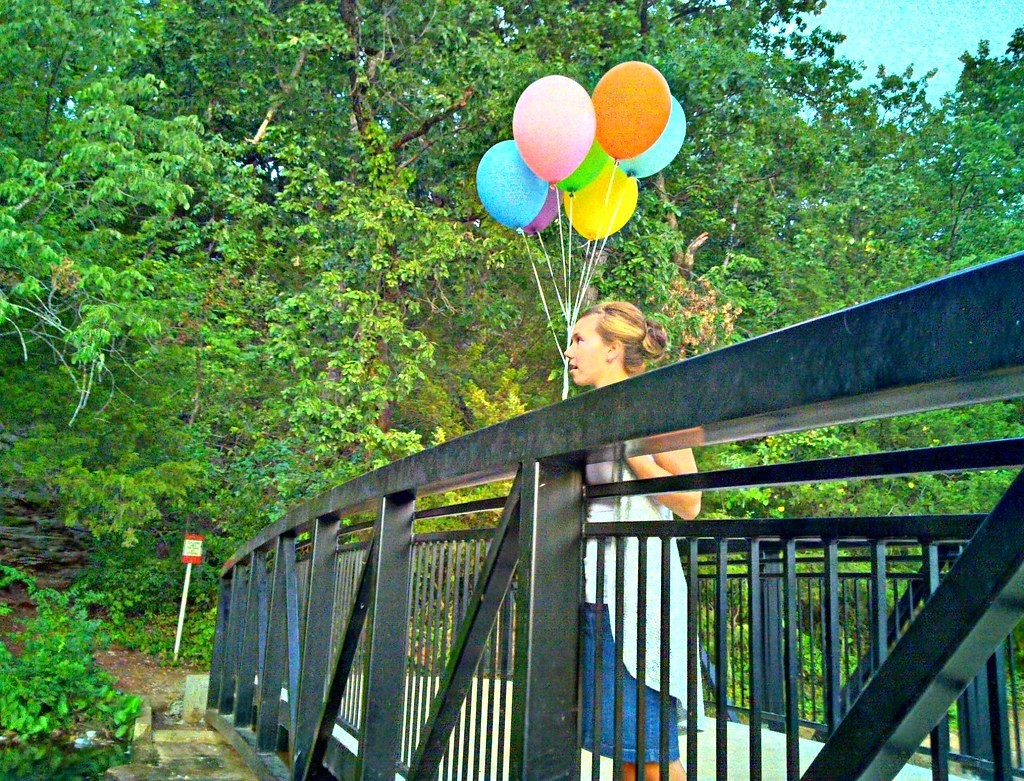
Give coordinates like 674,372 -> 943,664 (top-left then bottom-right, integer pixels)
591,62 -> 671,164
563,157 -> 638,240
557,137 -> 609,197
476,140 -> 549,235
522,186 -> 562,235
512,75 -> 596,189
620,96 -> 686,178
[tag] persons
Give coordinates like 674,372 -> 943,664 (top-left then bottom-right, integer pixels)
565,302 -> 701,781
